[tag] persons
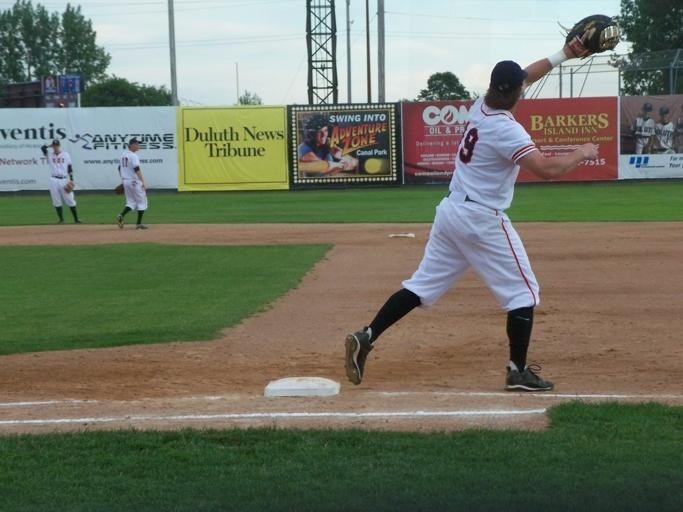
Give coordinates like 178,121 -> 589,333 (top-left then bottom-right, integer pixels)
116,139 -> 149,229
652,106 -> 677,155
297,116 -> 358,179
40,139 -> 84,224
628,101 -> 656,155
345,15 -> 621,392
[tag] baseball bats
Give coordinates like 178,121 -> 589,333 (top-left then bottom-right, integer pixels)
299,161 -> 343,172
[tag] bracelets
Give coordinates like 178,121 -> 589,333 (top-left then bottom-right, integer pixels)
546,49 -> 569,70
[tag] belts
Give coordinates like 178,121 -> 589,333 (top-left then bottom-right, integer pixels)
52,176 -> 67,179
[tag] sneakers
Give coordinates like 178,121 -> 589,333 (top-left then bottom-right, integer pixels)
504,363 -> 553,392
344,325 -> 374,385
117,214 -> 125,229
57,219 -> 63,223
75,219 -> 80,223
136,224 -> 148,229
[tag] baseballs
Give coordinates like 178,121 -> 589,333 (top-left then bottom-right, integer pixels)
365,158 -> 381,172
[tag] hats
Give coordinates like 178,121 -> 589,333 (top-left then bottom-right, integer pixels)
641,103 -> 653,112
659,106 -> 670,116
53,140 -> 59,145
490,61 -> 528,92
129,137 -> 142,144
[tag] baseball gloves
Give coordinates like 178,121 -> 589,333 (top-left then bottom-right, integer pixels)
115,184 -> 124,195
567,15 -> 620,59
64,183 -> 74,192
